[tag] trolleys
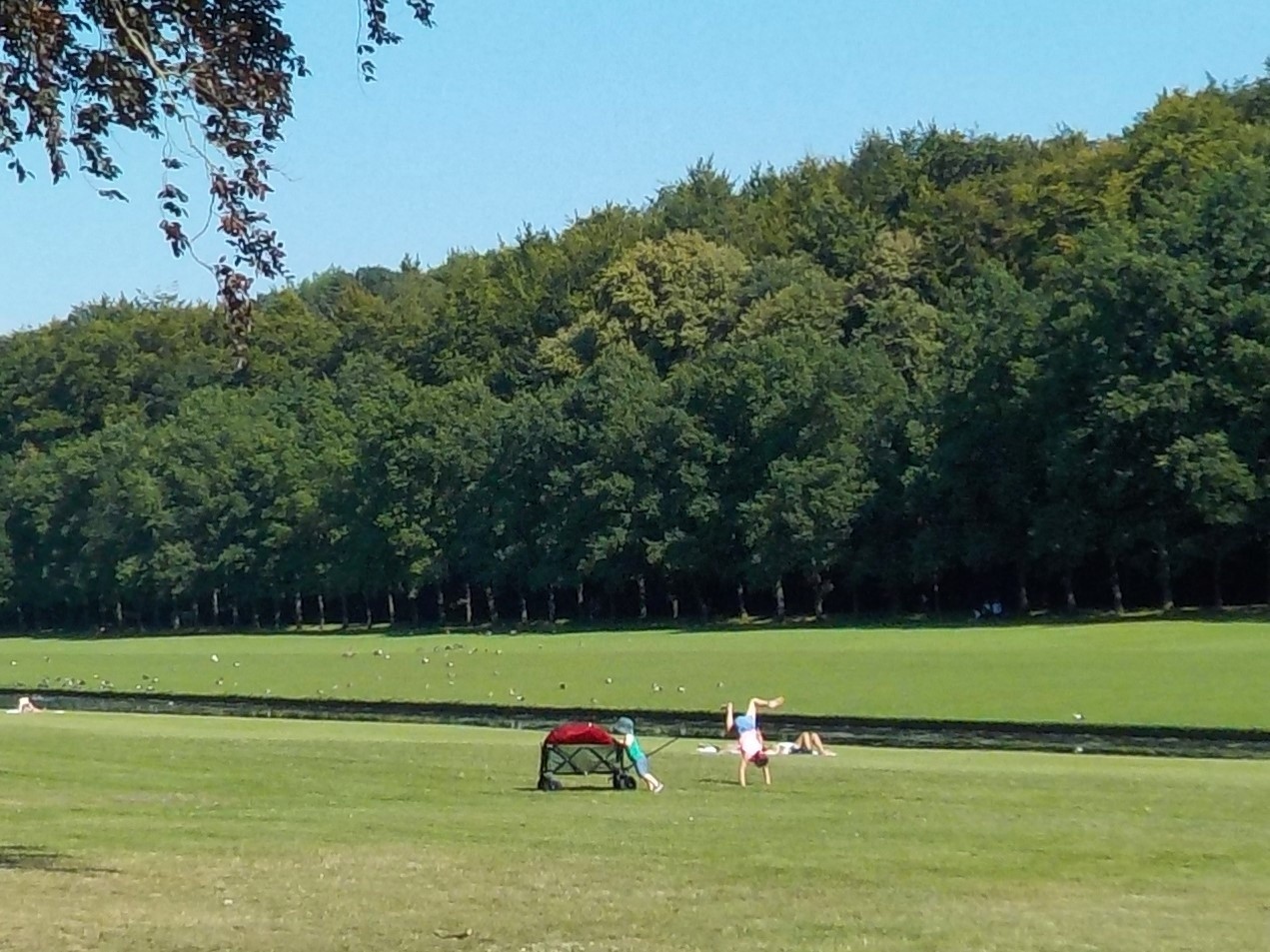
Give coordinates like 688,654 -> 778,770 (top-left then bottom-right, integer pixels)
536,738 -> 679,793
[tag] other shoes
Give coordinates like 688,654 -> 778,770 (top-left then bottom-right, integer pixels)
652,783 -> 664,793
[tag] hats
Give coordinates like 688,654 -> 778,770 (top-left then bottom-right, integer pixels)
613,717 -> 635,735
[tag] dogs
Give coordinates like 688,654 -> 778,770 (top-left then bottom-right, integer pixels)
3,695 -> 48,715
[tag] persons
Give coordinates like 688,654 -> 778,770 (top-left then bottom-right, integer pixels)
759,730 -> 837,757
606,717 -> 664,794
720,695 -> 784,789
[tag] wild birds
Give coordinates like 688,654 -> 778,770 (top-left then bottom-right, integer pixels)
315,642 -> 726,703
8,651 -> 272,694
1070,709 -> 1085,721
